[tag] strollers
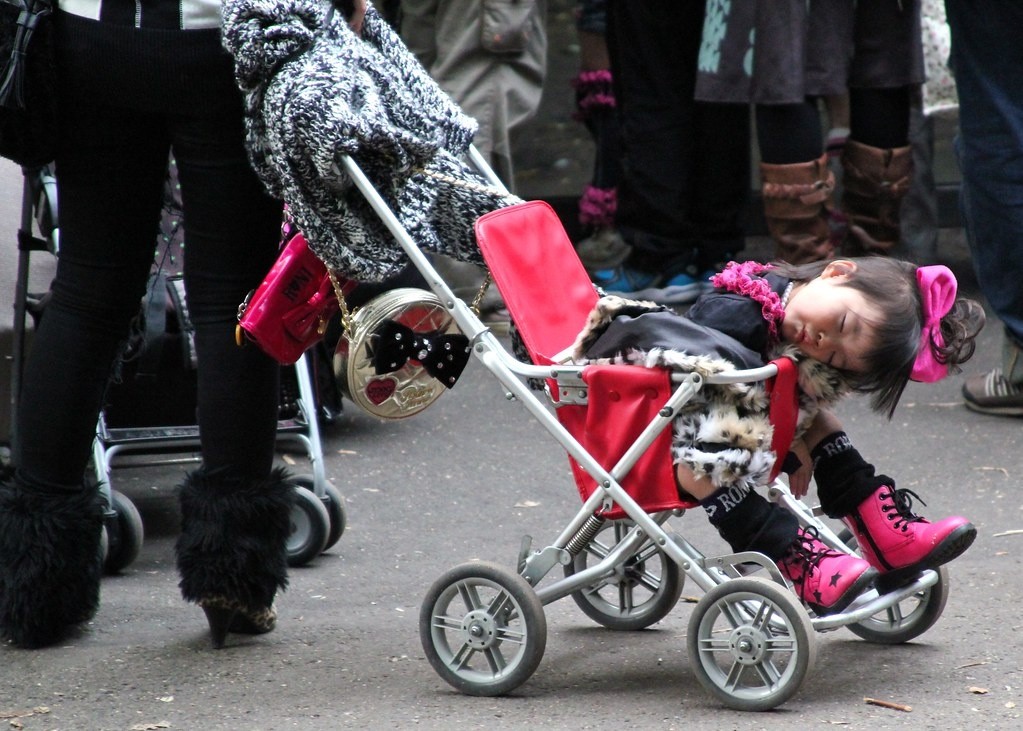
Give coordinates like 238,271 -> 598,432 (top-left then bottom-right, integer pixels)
0,148 -> 345,575
338,143 -> 949,712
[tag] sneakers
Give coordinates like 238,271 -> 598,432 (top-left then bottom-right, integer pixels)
701,268 -> 721,297
592,260 -> 701,304
963,366 -> 1023,418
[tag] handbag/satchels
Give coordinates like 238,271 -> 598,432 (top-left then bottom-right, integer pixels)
237,234 -> 353,366
331,285 -> 473,417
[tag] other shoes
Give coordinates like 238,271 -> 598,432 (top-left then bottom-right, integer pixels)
940,242 -> 980,289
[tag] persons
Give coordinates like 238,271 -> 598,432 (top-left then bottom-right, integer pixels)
692,1 -> 927,263
672,254 -> 984,617
944,1 -> 1022,414
0,0 -> 296,645
579,0 -> 747,306
386,1 -> 548,335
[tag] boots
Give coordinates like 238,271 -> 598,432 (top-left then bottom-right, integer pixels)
177,466 -> 297,647
773,531 -> 880,615
0,472 -> 106,649
838,134 -> 908,259
757,152 -> 839,266
845,484 -> 977,595
